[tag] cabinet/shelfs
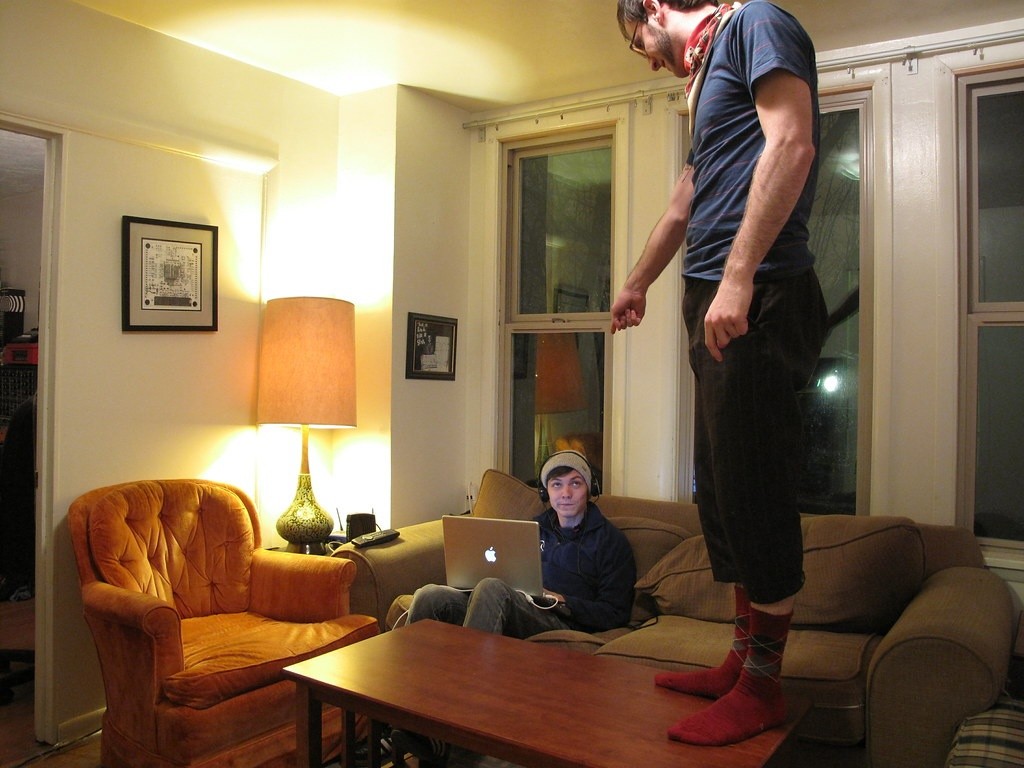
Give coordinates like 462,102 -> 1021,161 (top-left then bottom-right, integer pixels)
0,364 -> 38,426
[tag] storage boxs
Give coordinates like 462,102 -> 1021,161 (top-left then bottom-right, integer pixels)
4,343 -> 38,365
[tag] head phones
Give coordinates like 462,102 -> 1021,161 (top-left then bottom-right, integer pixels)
538,451 -> 600,502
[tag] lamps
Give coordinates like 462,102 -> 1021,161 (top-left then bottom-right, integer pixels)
257,297 -> 358,555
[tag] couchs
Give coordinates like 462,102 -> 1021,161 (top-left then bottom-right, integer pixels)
945,695 -> 1024,768
67,479 -> 386,768
330,494 -> 1024,768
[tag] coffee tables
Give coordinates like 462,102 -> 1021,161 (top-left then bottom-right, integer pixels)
282,619 -> 813,768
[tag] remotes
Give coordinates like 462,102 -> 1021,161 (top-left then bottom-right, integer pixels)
351,529 -> 401,547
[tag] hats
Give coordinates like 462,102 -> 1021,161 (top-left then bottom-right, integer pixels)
541,450 -> 591,499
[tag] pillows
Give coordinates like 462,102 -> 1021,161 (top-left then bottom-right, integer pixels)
475,469 -> 692,619
634,514 -> 926,634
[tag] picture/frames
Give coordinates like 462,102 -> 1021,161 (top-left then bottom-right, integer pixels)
405,312 -> 458,381
123,216 -> 219,331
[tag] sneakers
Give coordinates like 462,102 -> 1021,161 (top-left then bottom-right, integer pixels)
353,723 -> 393,767
391,729 -> 451,768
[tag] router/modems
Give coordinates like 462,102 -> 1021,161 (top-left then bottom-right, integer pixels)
346,513 -> 376,542
328,508 -> 374,542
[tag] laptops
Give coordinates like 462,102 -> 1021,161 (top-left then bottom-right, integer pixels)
442,516 -> 558,606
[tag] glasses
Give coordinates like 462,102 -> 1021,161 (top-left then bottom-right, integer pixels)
630,19 -> 647,58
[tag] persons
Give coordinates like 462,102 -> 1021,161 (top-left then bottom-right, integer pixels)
607,0 -> 827,748
371,449 -> 636,764
